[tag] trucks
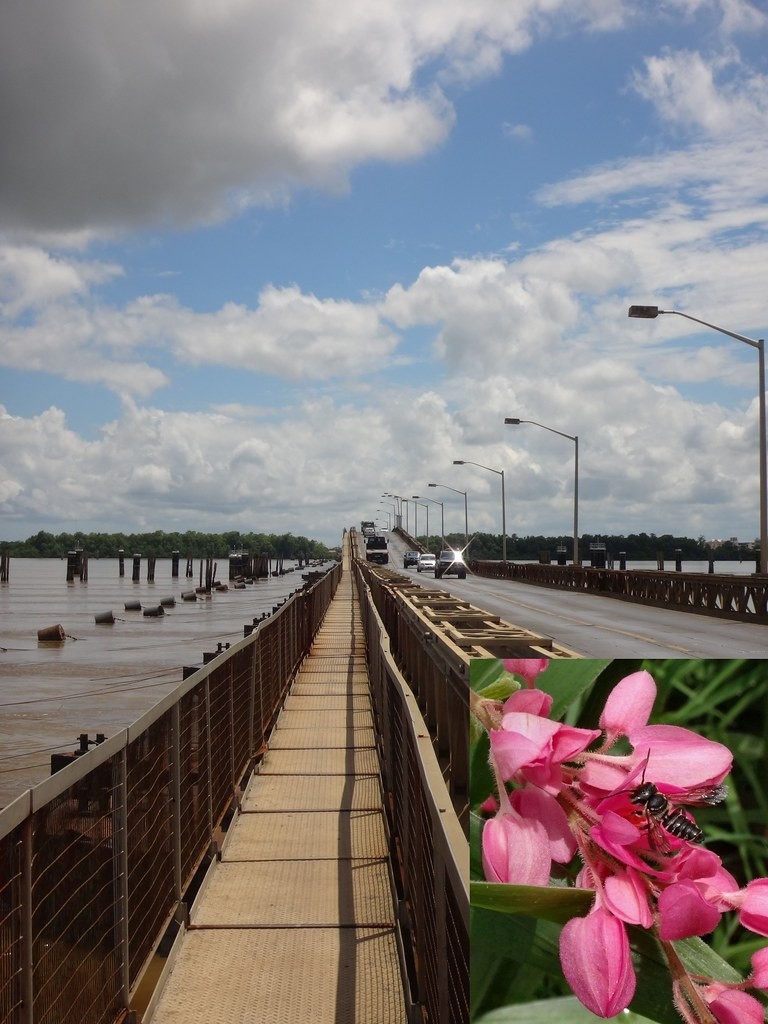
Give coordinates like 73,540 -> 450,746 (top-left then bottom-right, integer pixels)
361,521 -> 375,533
364,536 -> 389,564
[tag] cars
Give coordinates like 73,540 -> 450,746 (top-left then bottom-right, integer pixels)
417,554 -> 436,572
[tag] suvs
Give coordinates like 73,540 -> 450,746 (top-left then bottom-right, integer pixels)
364,528 -> 375,537
435,551 -> 466,579
404,551 -> 420,569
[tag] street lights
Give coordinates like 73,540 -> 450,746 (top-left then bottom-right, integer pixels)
428,483 -> 469,557
504,417 -> 579,565
452,461 -> 507,564
376,493 -> 408,532
402,499 -> 428,549
627,305 -> 768,579
